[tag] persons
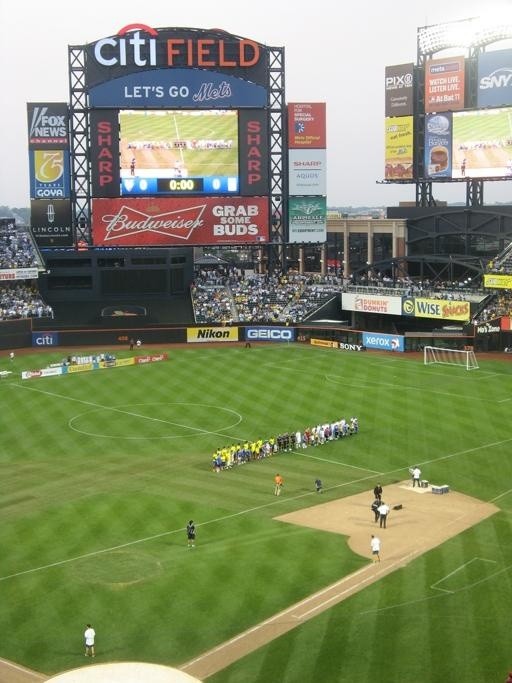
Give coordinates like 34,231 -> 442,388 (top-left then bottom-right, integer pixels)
83,622 -> 97,658
128,139 -> 233,152
410,465 -> 423,489
188,263 -> 340,325
378,501 -> 388,530
60,352 -> 114,366
374,484 -> 384,498
460,156 -> 467,174
136,338 -> 143,350
129,157 -> 136,175
1,229 -> 56,321
185,518 -> 196,549
313,477 -> 324,494
368,534 -> 383,566
8,350 -> 16,363
210,416 -> 363,474
349,270 -> 472,301
273,473 -> 284,496
370,496 -> 383,523
472,260 -> 512,330
129,337 -> 135,350
